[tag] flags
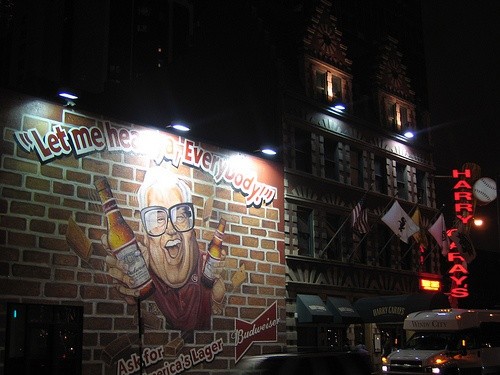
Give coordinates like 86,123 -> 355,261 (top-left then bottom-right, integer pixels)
410,205 -> 422,245
428,211 -> 449,256
380,200 -> 420,243
353,192 -> 370,234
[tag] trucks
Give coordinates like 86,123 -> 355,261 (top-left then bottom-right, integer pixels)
381,308 -> 500,375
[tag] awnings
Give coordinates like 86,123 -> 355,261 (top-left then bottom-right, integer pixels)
362,295 -> 411,324
296,293 -> 334,324
326,298 -> 361,327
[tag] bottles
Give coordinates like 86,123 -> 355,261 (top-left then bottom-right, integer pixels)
200,218 -> 227,288
94,176 -> 156,301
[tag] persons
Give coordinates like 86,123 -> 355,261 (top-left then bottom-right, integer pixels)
101,168 -> 226,330
